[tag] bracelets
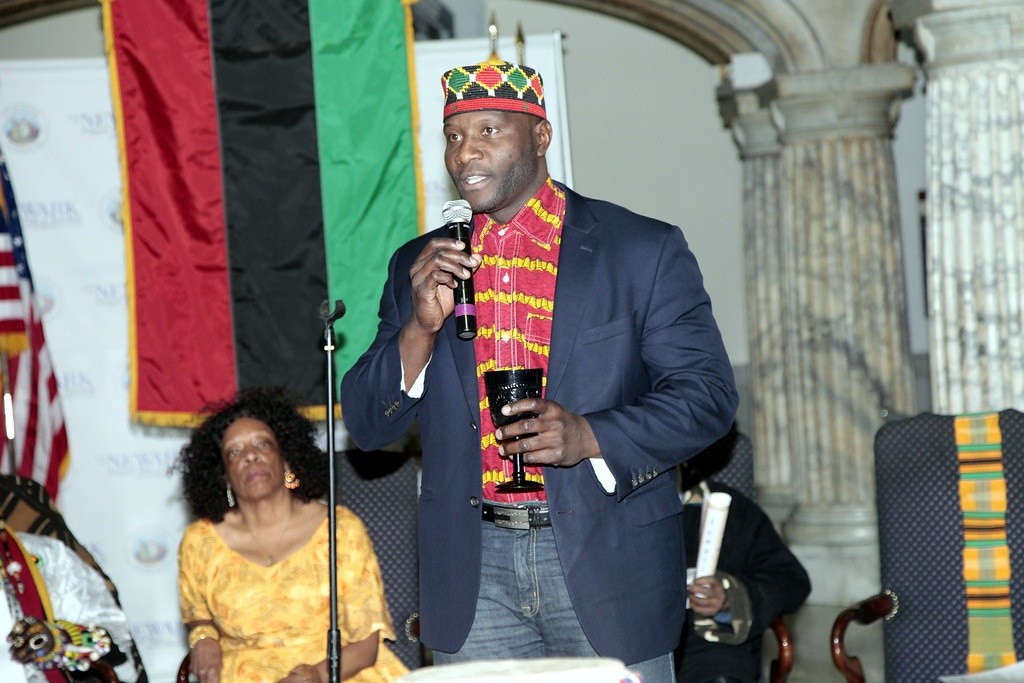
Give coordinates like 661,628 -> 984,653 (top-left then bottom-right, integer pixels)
90,626 -> 111,661
721,578 -> 731,590
188,624 -> 219,648
33,621 -> 61,669
79,625 -> 92,671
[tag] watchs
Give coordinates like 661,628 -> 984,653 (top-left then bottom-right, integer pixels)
57,620 -> 80,670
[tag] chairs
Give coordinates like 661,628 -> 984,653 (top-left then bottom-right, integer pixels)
177,449 -> 421,683
829,406 -> 1024,683
711,432 -> 796,682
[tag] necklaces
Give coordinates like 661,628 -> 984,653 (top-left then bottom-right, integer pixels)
251,513 -> 295,564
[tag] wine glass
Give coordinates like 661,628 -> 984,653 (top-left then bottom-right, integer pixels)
483,368 -> 545,490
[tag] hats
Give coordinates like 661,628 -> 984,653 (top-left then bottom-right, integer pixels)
439,52 -> 547,118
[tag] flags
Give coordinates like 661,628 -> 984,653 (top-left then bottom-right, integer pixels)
0,158 -> 68,499
100,0 -> 425,429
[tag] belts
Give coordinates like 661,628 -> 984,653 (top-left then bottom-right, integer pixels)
483,502 -> 552,531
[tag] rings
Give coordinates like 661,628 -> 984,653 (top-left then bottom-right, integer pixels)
29,634 -> 47,650
24,617 -> 36,624
13,636 -> 25,648
37,650 -> 45,657
30,624 -> 42,633
13,623 -> 24,633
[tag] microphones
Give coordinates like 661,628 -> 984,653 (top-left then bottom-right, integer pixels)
441,199 -> 477,339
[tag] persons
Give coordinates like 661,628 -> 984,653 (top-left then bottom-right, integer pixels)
341,65 -> 739,683
676,462 -> 812,683
0,530 -> 137,683
177,388 -> 410,683
